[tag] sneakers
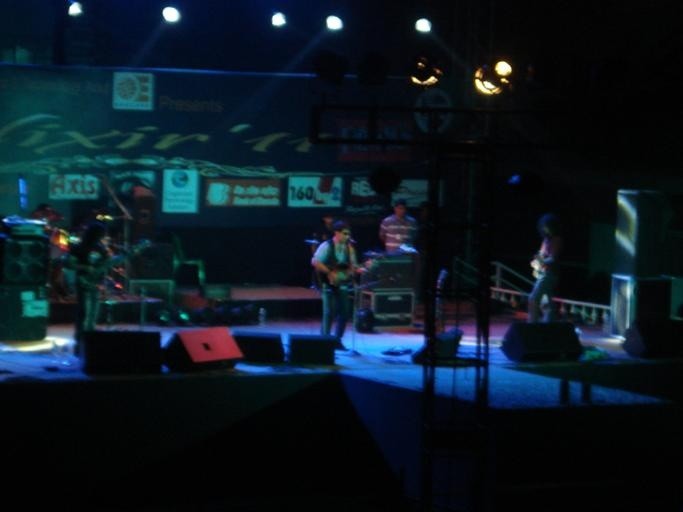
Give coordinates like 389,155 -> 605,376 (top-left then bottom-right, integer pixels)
336,342 -> 349,351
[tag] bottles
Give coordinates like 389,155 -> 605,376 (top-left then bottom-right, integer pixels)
259,308 -> 264,330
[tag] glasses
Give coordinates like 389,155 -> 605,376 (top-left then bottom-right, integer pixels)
340,231 -> 351,236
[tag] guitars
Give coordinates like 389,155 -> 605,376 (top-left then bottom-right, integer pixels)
79,239 -> 150,290
328,259 -> 379,286
530,254 -> 548,278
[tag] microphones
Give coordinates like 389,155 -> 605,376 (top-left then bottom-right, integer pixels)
348,237 -> 356,244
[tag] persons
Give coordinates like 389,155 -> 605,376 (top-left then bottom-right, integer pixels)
378,198 -> 419,255
311,220 -> 357,351
311,213 -> 336,289
415,200 -> 450,333
527,213 -> 583,324
30,199 -> 117,357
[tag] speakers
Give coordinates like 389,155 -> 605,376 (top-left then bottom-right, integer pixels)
164,326 -> 244,372
502,321 -> 582,362
236,333 -> 282,360
615,189 -> 667,277
622,319 -> 682,358
609,274 -> 672,338
0,239 -> 47,285
412,329 -> 463,365
287,334 -> 334,366
0,285 -> 46,341
81,330 -> 157,375
